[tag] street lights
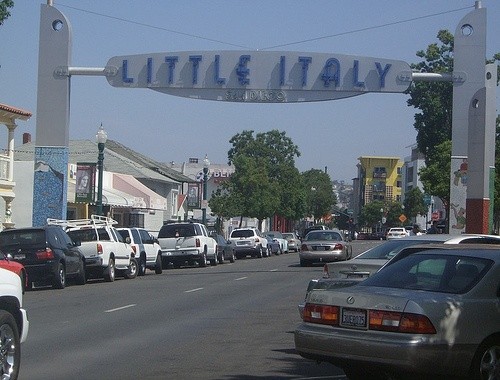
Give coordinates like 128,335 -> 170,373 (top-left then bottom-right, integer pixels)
201,153 -> 210,225
95,121 -> 107,215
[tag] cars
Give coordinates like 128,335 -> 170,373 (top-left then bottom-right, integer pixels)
386,228 -> 408,240
357,232 -> 386,240
282,233 -> 303,252
209,233 -> 235,263
262,235 -> 282,255
300,230 -> 352,265
295,244 -> 500,380
0,249 -> 28,294
262,232 -> 288,254
298,234 -> 500,319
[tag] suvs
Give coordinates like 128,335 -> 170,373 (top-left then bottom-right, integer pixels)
116,228 -> 162,276
0,267 -> 28,380
47,215 -> 139,282
0,227 -> 86,288
157,222 -> 218,268
229,228 -> 269,259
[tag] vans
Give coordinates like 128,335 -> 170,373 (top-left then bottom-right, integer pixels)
310,225 -> 332,239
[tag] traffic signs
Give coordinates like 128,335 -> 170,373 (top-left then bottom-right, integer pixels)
399,214 -> 408,222
